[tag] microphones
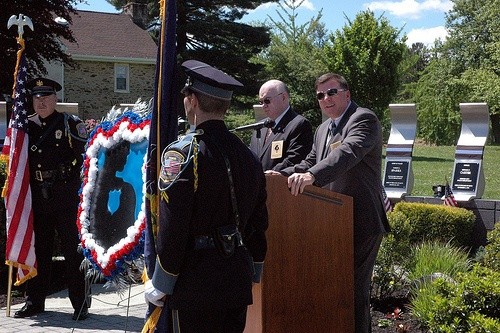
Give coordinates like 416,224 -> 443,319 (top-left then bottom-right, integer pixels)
236,118 -> 276,132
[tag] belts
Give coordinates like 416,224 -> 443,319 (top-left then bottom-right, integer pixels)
33,170 -> 60,182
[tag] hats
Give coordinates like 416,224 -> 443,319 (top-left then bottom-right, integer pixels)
30,77 -> 62,94
181,59 -> 244,100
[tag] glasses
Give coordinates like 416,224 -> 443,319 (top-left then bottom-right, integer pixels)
317,88 -> 343,100
259,92 -> 283,105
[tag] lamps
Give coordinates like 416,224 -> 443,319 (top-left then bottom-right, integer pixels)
432,185 -> 446,198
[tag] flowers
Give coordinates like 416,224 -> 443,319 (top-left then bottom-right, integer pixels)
77,110 -> 152,275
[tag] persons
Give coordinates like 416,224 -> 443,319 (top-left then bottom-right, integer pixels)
265,73 -> 391,333
250,80 -> 311,176
144,58 -> 269,333
13,77 -> 91,320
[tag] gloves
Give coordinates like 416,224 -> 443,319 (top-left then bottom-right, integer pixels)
143,279 -> 166,307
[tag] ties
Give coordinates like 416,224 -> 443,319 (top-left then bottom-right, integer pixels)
326,120 -> 336,146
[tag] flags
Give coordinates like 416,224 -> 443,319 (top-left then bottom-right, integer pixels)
380,185 -> 392,212
0,38 -> 38,285
141,0 -> 182,333
444,180 -> 457,207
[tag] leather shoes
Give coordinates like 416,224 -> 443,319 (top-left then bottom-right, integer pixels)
14,304 -> 45,318
73,308 -> 89,321
